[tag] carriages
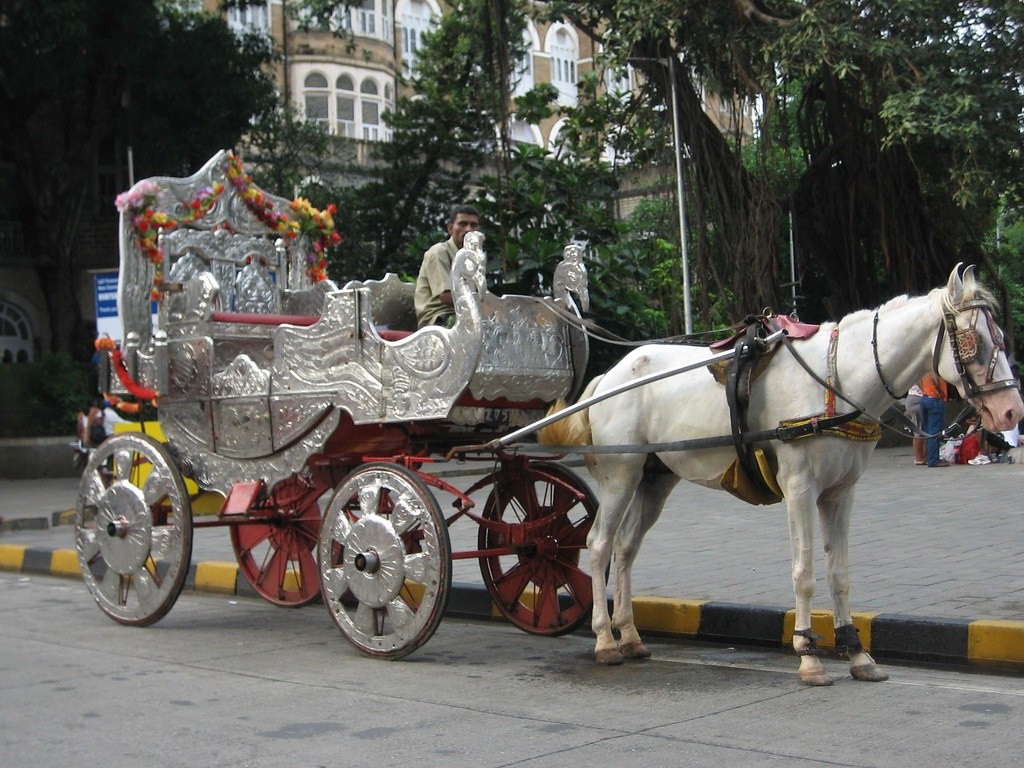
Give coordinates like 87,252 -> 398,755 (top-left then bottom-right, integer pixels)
69,148 -> 1024,689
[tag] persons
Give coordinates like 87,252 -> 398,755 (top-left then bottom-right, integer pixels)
968,422 -> 1019,466
414,205 -> 480,329
905,385 -> 928,465
919,372 -> 949,467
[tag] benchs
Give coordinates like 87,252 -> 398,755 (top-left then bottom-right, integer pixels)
155,226 -> 338,339
358,273 -> 417,340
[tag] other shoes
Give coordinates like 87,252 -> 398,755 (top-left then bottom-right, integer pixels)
968,453 -> 991,466
991,453 -> 998,462
934,460 -> 948,467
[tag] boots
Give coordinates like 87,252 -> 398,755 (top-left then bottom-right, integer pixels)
912,439 -> 927,465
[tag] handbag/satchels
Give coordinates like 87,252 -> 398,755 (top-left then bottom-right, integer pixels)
938,433 -> 964,464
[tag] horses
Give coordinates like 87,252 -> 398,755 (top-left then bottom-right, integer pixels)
539,262 -> 1024,686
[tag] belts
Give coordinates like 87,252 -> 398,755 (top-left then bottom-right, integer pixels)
924,396 -> 933,398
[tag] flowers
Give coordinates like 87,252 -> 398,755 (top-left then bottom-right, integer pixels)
115,153 -> 342,303
